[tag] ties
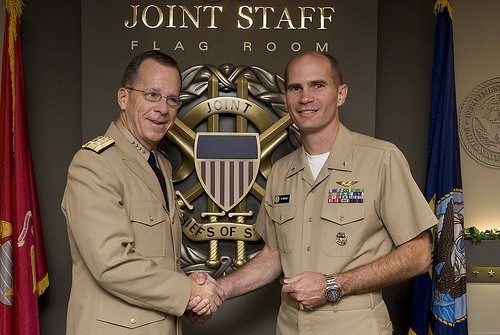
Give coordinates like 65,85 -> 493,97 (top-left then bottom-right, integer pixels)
147,152 -> 169,212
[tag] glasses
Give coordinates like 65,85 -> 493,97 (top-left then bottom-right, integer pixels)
125,87 -> 182,108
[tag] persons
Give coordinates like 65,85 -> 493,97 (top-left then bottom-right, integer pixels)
59,50 -> 226,335
186,49 -> 440,335
407,0 -> 469,335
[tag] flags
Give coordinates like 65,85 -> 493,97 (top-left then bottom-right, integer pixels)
0,0 -> 51,335
193,131 -> 261,213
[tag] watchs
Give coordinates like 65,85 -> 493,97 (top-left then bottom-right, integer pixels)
324,274 -> 342,303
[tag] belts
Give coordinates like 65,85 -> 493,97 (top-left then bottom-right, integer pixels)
281,288 -> 382,311
